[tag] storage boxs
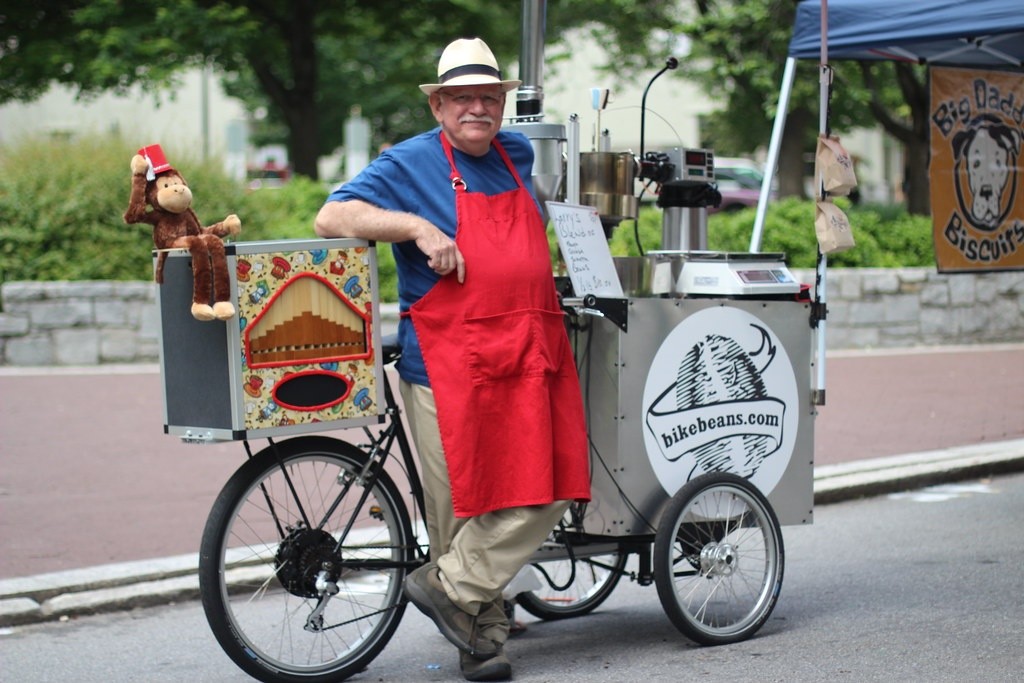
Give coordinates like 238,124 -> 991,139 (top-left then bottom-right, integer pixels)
150,240 -> 386,439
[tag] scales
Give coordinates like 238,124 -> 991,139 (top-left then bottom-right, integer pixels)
647,249 -> 800,299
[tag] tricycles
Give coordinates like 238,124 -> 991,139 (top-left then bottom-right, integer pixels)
197,296 -> 786,682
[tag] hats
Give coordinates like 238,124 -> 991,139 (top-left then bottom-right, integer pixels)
418,38 -> 523,96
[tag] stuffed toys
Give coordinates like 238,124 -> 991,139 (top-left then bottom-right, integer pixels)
124,154 -> 240,321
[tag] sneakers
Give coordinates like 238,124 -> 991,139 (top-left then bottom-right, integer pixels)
458,642 -> 511,681
402,561 -> 496,658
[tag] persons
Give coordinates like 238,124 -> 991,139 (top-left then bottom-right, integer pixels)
314,37 -> 591,683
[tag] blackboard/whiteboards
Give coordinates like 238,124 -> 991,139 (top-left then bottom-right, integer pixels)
545,201 -> 626,299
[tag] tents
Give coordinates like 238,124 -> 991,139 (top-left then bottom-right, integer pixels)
748,0 -> 1024,255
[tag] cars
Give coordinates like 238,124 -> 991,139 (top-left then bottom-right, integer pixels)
707,160 -> 764,212
